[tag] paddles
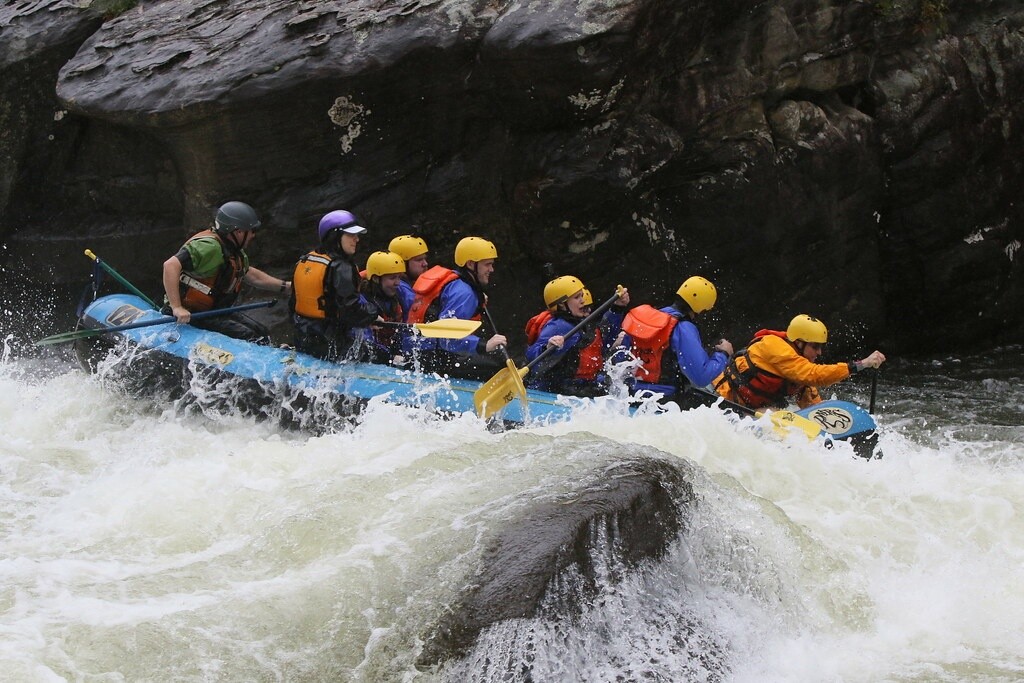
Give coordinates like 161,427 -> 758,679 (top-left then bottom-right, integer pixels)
36,298 -> 278,346
483,304 -> 527,406
690,387 -> 822,443
869,368 -> 879,414
84,249 -> 161,311
473,285 -> 624,418
370,318 -> 482,339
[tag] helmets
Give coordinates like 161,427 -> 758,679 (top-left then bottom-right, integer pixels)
787,314 -> 827,343
676,275 -> 717,314
318,210 -> 356,244
455,236 -> 498,267
544,275 -> 585,312
214,201 -> 260,234
583,288 -> 594,306
388,235 -> 428,260
366,250 -> 406,280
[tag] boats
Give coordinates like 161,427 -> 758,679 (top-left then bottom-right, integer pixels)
72,293 -> 880,466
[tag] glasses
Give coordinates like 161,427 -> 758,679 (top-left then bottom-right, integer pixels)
808,343 -> 824,350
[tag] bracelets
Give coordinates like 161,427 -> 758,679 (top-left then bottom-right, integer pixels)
856,360 -> 863,371
281,281 -> 286,292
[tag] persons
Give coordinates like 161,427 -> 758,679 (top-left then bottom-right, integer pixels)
359,233 -> 428,353
162,202 -> 294,346
711,314 -> 887,414
404,237 -> 507,381
524,275 -> 629,391
290,210 -> 384,363
620,276 -> 733,390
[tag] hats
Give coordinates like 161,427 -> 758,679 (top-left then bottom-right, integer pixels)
341,224 -> 367,234
